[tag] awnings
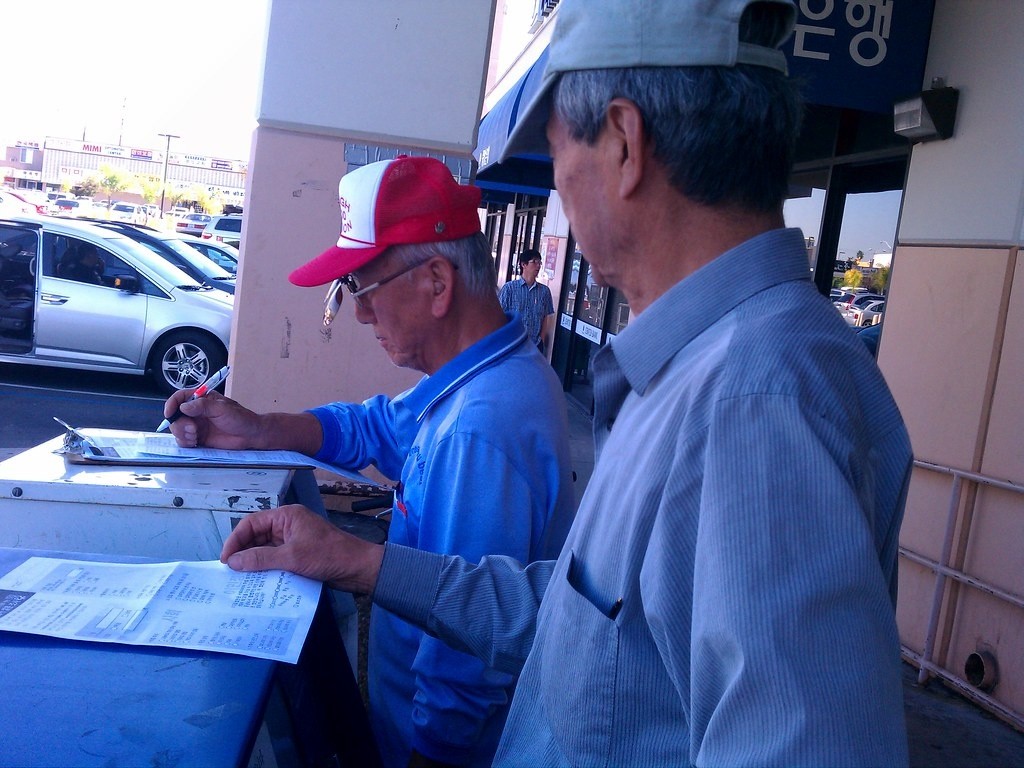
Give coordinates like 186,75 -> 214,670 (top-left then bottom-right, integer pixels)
473,0 -> 932,209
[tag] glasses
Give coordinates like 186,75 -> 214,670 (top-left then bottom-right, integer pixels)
340,254 -> 457,311
528,259 -> 542,264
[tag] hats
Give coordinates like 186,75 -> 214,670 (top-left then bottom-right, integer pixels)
288,153 -> 480,287
497,0 -> 798,163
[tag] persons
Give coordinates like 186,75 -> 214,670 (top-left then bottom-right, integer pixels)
219,0 -> 914,768
498,248 -> 553,354
166,156 -> 575,768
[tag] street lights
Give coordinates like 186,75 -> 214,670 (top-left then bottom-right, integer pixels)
157,132 -> 180,218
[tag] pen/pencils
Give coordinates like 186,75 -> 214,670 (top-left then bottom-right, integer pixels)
156,365 -> 231,433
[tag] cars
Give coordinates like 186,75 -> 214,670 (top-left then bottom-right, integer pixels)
0,187 -> 242,296
829,286 -> 886,329
0,216 -> 234,399
